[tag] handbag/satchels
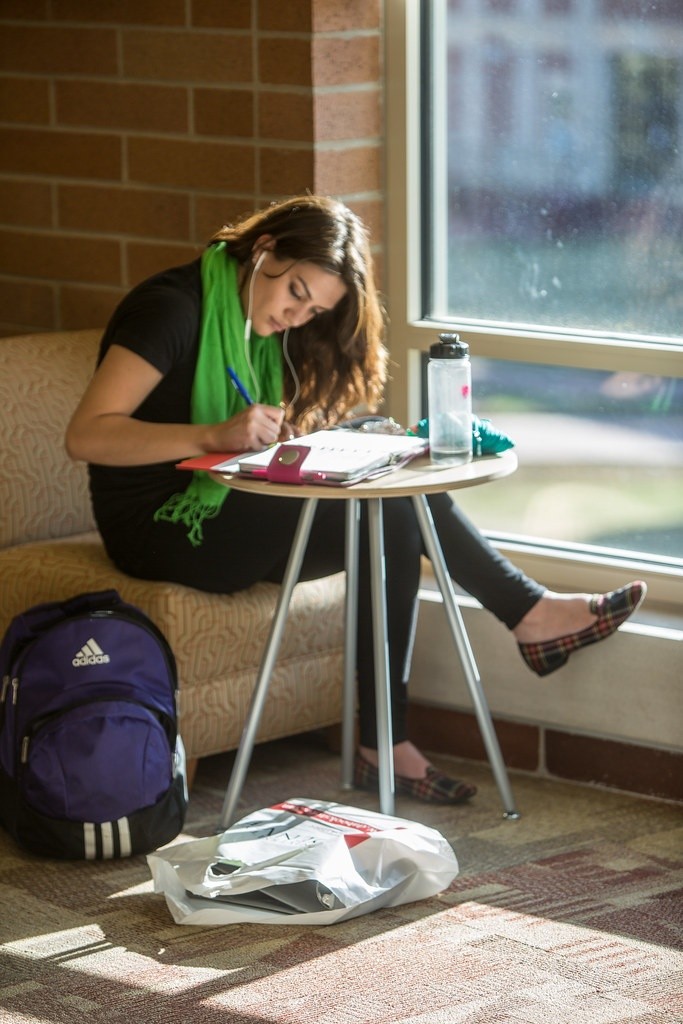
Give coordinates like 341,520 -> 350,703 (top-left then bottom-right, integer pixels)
145,797 -> 458,925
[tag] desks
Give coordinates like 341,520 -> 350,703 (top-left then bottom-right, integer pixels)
205,443 -> 518,822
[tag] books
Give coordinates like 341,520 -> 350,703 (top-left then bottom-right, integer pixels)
239,428 -> 429,487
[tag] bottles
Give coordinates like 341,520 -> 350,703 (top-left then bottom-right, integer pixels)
427,332 -> 475,468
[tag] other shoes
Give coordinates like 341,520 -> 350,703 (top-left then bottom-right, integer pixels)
518,580 -> 648,676
352,747 -> 478,805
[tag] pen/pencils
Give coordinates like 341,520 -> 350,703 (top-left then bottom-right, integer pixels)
225,366 -> 253,408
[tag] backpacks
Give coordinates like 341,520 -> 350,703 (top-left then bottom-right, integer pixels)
1,586 -> 189,864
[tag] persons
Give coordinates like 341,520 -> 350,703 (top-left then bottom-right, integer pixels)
62,196 -> 646,807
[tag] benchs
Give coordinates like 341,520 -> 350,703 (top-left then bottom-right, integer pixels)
0,321 -> 347,794
